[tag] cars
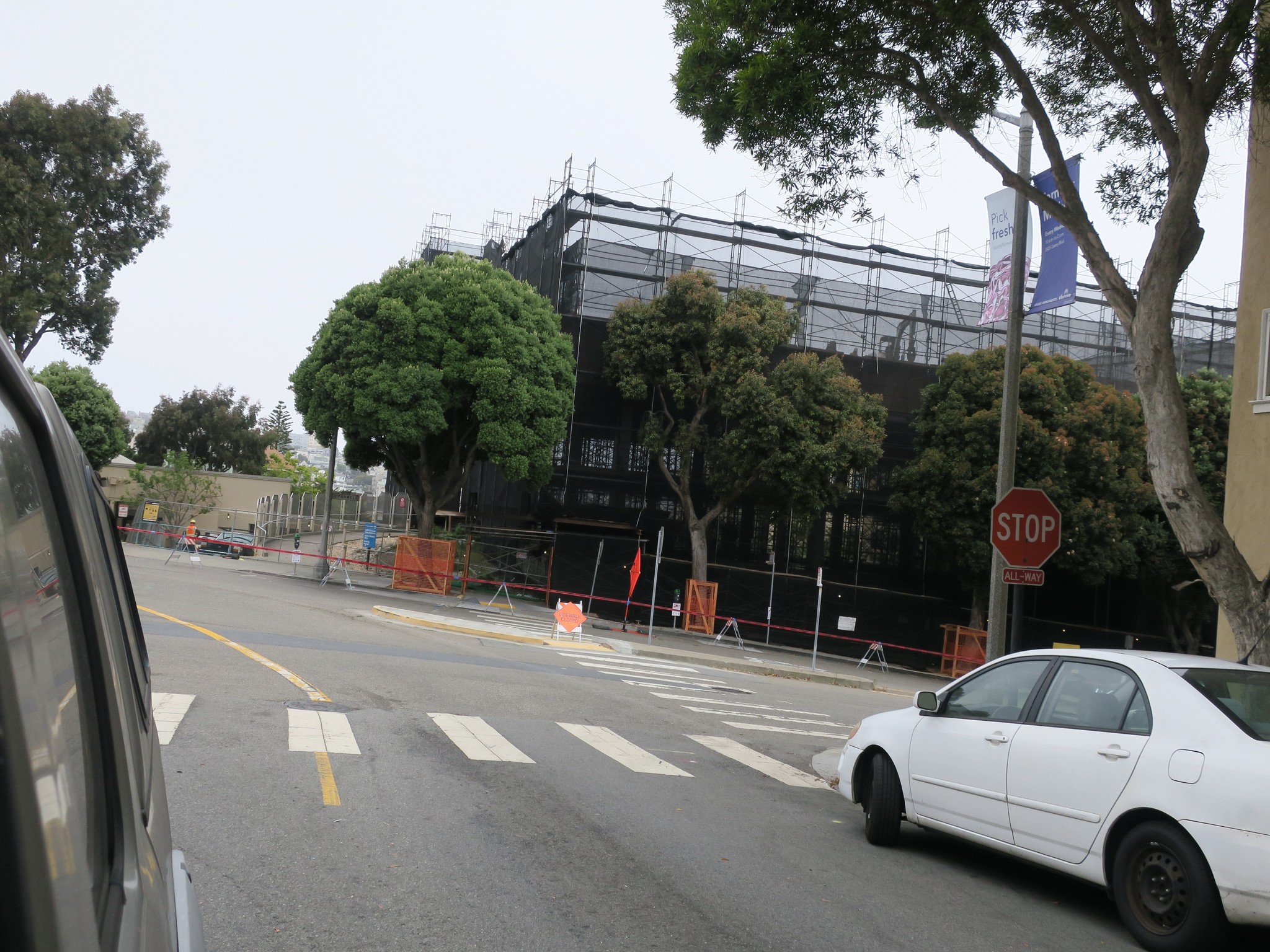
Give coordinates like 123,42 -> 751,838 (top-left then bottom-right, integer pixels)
0,327 -> 204,952
837,648 -> 1270,952
40,571 -> 61,597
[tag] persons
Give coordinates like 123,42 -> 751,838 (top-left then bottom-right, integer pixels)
183,519 -> 200,553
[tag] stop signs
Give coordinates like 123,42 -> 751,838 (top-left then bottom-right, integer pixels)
991,488 -> 1061,568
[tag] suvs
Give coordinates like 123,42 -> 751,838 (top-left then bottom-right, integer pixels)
181,531 -> 255,557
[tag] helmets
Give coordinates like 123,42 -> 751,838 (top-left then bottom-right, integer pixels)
190,520 -> 195,523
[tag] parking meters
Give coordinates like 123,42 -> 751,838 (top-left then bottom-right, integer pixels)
292,534 -> 300,575
